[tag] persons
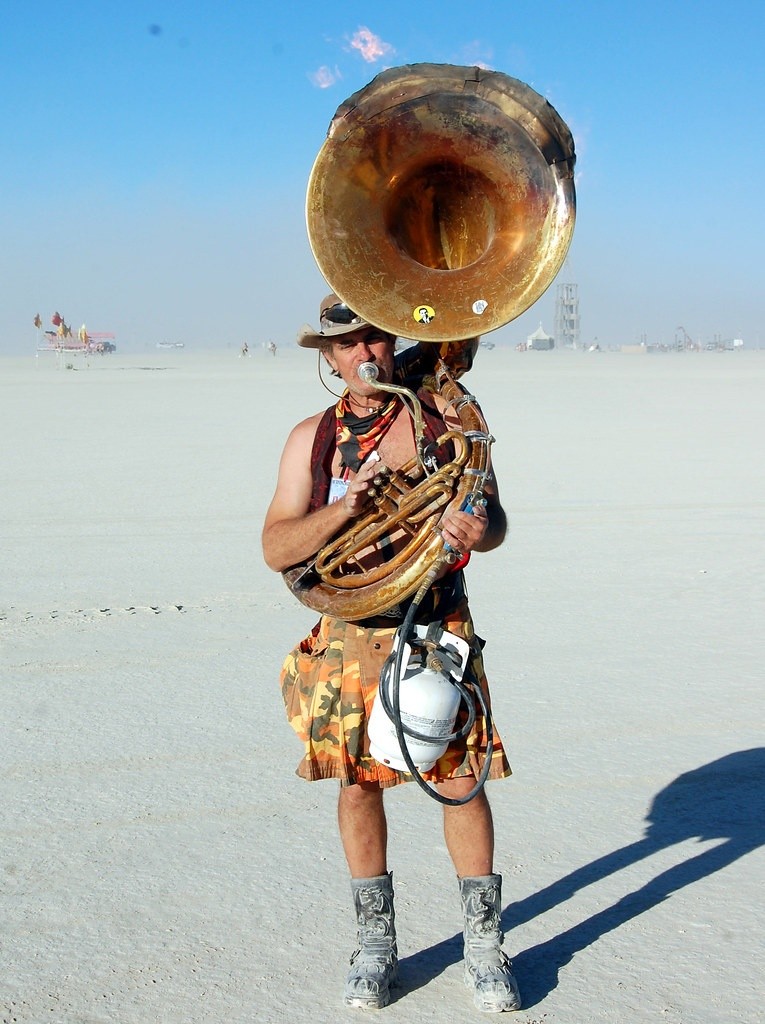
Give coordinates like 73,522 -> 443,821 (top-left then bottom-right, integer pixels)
262,289 -> 522,1014
269,342 -> 275,355
241,343 -> 248,356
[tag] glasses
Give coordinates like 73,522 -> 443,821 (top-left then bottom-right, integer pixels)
320,303 -> 358,324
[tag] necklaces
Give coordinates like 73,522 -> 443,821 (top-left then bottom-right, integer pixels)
350,395 -> 376,413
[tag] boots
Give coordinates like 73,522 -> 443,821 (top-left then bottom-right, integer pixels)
344,871 -> 398,1009
456,874 -> 522,1013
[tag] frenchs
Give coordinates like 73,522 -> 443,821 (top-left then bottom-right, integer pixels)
281,61 -> 579,621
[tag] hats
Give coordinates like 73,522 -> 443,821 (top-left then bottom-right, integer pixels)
296,294 -> 372,348
